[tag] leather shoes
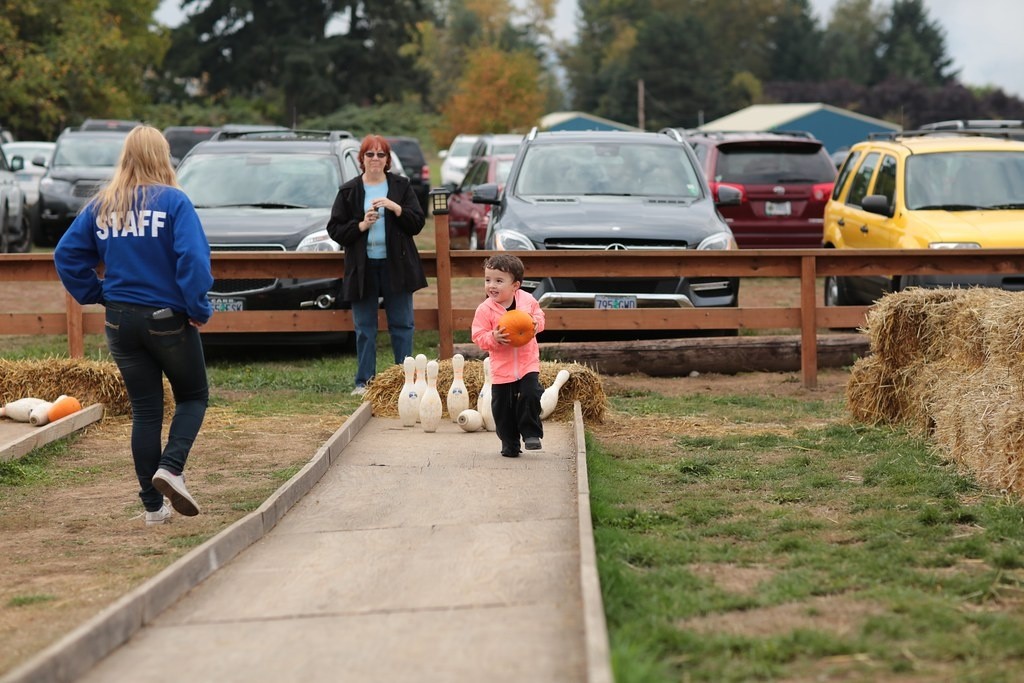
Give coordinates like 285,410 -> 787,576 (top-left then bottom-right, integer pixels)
524,435 -> 541,449
501,440 -> 521,457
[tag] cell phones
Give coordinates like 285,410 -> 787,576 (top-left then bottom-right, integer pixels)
153,308 -> 173,319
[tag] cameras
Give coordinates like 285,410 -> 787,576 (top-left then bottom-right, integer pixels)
372,207 -> 379,220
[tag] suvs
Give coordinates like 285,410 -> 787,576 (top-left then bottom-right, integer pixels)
382,137 -> 429,216
821,128 -> 1024,329
681,130 -> 839,250
171,130 -> 363,346
33,125 -> 129,250
471,125 -> 743,342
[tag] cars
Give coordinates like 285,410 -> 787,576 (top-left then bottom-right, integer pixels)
0,146 -> 33,253
448,154 -> 516,249
464,132 -> 525,171
0,142 -> 57,206
80,119 -> 145,133
162,127 -> 219,166
436,133 -> 478,190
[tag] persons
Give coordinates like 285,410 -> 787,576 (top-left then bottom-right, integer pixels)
55,125 -> 214,526
471,255 -> 545,458
326,135 -> 428,397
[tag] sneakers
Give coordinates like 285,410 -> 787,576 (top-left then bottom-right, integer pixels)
129,505 -> 171,526
351,385 -> 366,396
152,467 -> 200,517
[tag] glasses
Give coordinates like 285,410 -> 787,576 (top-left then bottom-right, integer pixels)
365,152 -> 385,158
